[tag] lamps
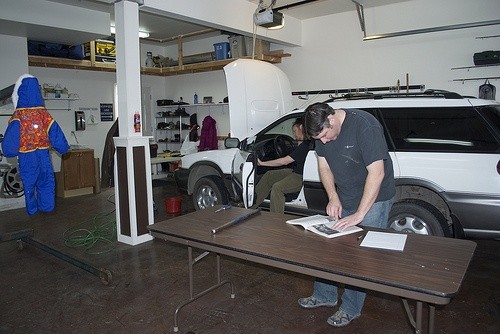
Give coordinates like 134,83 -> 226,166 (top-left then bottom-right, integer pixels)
111,27 -> 150,38
256,8 -> 284,30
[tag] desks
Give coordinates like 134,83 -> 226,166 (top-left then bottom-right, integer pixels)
146,205 -> 477,334
151,153 -> 184,180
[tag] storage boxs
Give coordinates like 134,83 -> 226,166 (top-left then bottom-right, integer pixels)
227,36 -> 246,57
213,42 -> 230,60
245,36 -> 270,55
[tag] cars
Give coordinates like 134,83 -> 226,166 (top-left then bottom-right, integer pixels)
173,57 -> 500,241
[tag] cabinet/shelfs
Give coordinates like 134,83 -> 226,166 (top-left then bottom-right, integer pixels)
44,98 -> 80,111
155,103 -> 229,148
451,35 -> 500,84
54,149 -> 95,199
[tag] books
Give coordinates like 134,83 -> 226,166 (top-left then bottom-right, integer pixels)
284,215 -> 364,239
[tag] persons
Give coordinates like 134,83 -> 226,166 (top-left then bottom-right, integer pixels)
245,117 -> 315,215
296,100 -> 397,326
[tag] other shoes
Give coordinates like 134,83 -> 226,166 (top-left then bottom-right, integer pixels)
298,295 -> 338,308
327,309 -> 361,327
